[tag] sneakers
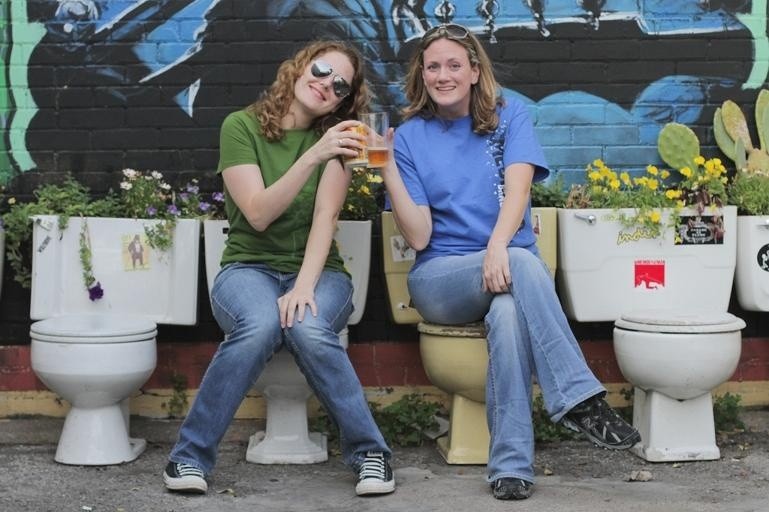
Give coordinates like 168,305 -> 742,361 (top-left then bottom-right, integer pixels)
489,477 -> 535,500
353,450 -> 396,498
560,395 -> 643,452
162,457 -> 209,495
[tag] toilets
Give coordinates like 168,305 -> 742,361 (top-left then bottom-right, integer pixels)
557,205 -> 747,464
737,214 -> 769,311
381,208 -> 556,464
26,213 -> 203,468
203,220 -> 373,466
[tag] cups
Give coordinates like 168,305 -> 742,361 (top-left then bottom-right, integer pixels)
357,111 -> 390,168
343,125 -> 368,168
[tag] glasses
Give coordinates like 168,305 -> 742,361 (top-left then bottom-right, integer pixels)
310,57 -> 352,98
422,24 -> 471,42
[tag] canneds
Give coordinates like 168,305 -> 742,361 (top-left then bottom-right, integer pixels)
340,123 -> 367,169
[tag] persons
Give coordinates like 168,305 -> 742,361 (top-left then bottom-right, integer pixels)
127,234 -> 146,270
161,37 -> 396,497
358,21 -> 644,500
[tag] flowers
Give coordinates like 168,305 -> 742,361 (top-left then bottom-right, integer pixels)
0,169 -> 201,301
557,157 -> 729,245
197,167 -> 384,220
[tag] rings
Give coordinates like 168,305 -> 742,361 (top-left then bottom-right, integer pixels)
338,139 -> 343,147
